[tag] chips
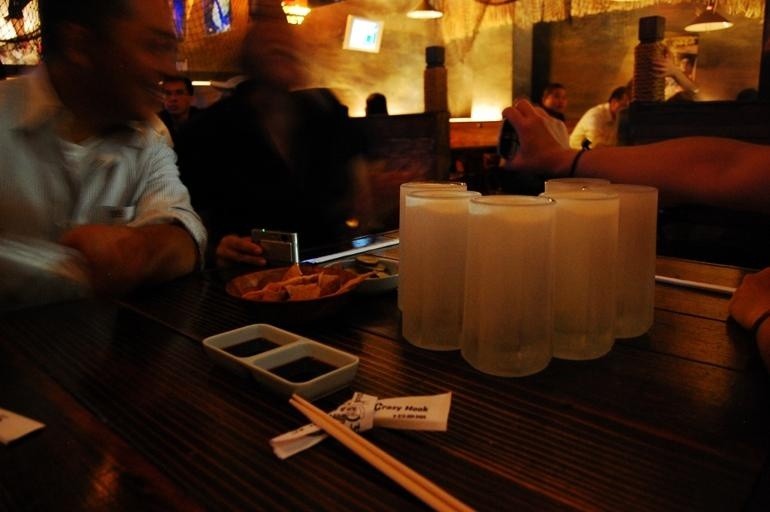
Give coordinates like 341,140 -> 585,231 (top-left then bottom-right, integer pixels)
243,264 -> 378,301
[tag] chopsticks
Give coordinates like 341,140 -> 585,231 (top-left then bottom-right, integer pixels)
288,393 -> 478,512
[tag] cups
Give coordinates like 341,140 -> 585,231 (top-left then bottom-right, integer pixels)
611,177 -> 657,341
399,177 -> 482,353
464,179 -> 619,374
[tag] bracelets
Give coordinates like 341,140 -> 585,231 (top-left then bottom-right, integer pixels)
747,309 -> 770,345
568,137 -> 592,179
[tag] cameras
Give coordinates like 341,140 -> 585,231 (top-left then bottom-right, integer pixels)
498,100 -> 566,162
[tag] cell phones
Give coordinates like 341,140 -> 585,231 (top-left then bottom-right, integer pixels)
252,229 -> 300,269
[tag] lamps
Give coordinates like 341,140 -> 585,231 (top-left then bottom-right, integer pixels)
280,0 -> 312,25
405,0 -> 445,20
684,0 -> 734,33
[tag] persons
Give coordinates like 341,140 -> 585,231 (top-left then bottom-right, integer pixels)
0,1 -> 207,282
160,1 -> 400,270
497,98 -> 769,369
494,52 -> 766,169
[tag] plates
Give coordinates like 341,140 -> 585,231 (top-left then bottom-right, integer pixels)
224,264 -> 360,304
323,256 -> 399,293
